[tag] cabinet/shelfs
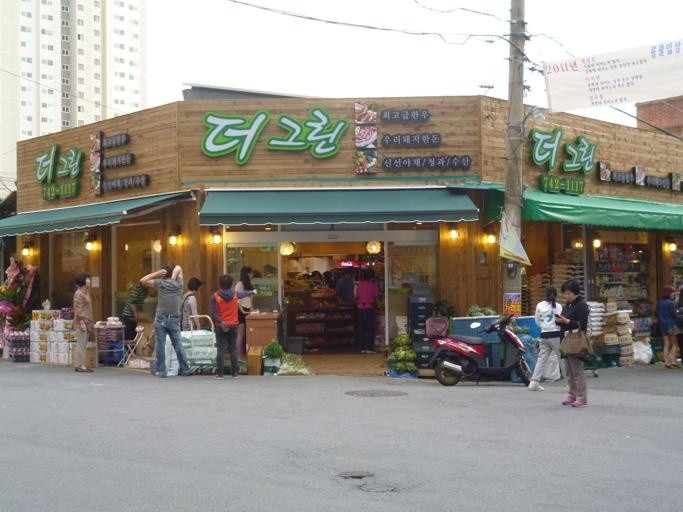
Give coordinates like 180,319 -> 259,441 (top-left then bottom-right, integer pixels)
594,258 -> 651,337
283,287 -> 356,348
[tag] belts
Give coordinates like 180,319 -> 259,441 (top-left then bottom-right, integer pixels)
166,315 -> 180,318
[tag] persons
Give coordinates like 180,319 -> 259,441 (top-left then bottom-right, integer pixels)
235,266 -> 254,362
631,287 -> 654,314
303,265 -> 378,355
70,271 -> 95,373
553,279 -> 591,407
527,285 -> 569,392
655,284 -> 681,370
121,282 -> 149,354
140,263 -> 196,377
210,274 -> 258,380
181,278 -> 203,330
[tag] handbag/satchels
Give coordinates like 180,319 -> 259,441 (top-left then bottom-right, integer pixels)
558,332 -> 593,362
650,323 -> 661,337
673,325 -> 683,335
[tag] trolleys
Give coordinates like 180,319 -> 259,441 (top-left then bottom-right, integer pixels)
583,358 -> 599,378
188,314 -> 249,377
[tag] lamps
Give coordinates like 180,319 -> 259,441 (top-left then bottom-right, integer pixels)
22,240 -> 34,256
448,223 -> 459,239
83,231 -> 96,251
168,225 -> 181,245
213,227 -> 221,244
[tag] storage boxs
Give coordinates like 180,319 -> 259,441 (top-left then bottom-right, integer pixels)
29,309 -> 95,368
597,311 -> 634,366
409,285 -> 540,384
248,346 -> 262,375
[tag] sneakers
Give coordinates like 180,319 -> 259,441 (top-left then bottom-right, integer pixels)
665,363 -> 681,369
75,366 -> 93,371
528,380 -> 588,408
361,350 -> 376,354
215,375 -> 223,379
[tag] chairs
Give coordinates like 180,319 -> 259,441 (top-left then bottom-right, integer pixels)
116,326 -> 145,368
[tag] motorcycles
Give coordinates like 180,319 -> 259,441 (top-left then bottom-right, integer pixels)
425,298 -> 532,387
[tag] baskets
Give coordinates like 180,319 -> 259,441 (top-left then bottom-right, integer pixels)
409,285 -> 441,369
